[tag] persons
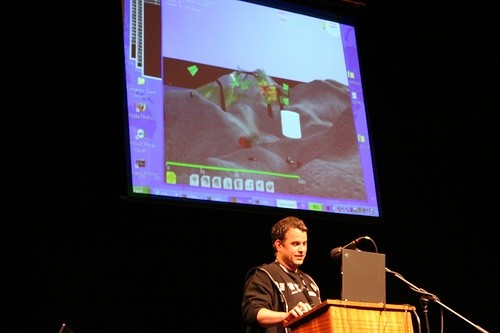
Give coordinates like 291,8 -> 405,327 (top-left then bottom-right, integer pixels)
242,216 -> 322,333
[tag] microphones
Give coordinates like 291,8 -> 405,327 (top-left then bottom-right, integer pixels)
330,236 -> 365,258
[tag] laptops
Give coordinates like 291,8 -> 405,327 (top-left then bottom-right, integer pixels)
341,249 -> 386,303
